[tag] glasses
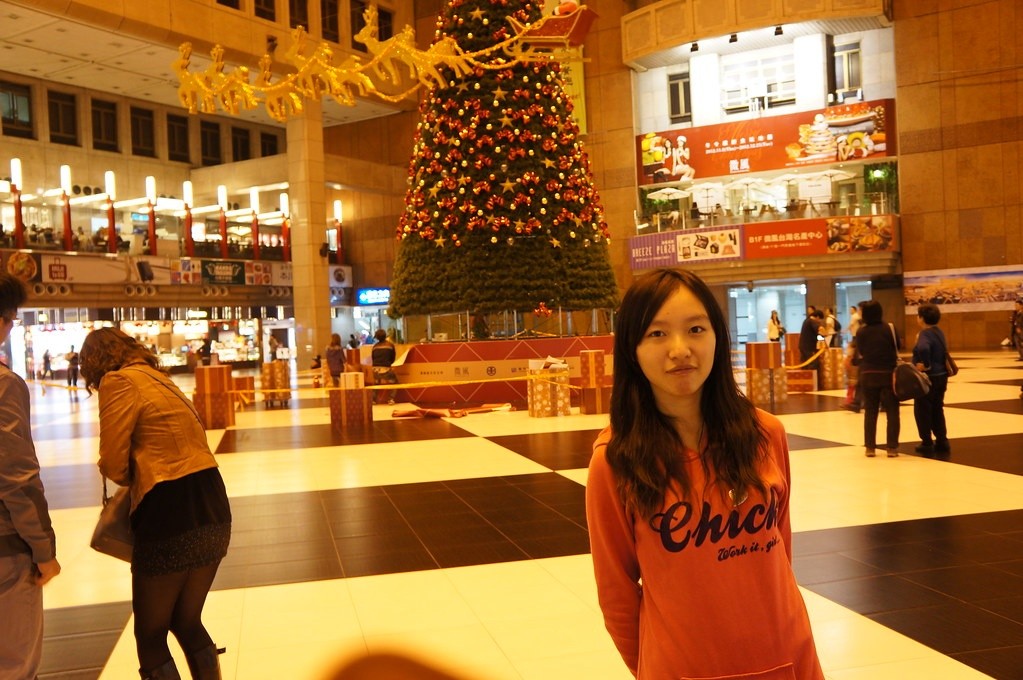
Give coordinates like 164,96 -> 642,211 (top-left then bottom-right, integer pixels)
0,316 -> 21,327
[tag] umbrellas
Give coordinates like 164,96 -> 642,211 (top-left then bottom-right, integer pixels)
647,169 -> 856,210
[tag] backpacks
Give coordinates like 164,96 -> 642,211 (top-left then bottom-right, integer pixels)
827,316 -> 841,332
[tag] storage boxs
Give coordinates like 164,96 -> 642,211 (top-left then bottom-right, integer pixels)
745,333 -> 843,405
527,349 -> 613,418
191,362 -> 291,427
328,373 -> 371,427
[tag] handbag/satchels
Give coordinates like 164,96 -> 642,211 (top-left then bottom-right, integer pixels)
945,352 -> 961,377
779,328 -> 786,337
893,359 -> 932,402
90,477 -> 135,563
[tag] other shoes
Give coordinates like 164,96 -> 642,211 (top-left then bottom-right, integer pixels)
388,400 -> 395,404
887,448 -> 899,456
914,441 -> 936,454
865,447 -> 875,456
937,441 -> 951,452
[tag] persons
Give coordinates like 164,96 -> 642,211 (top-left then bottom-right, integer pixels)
142,231 -> 149,244
0,224 -> 124,252
799,305 -> 858,373
365,332 -> 375,344
64,345 -> 78,386
42,349 -> 54,380
325,333 -> 346,385
349,334 -> 360,348
852,301 -> 900,456
1011,301 -> 1023,361
228,238 -> 252,252
668,208 -> 679,222
690,202 -> 700,218
80,328 -> 231,680
768,310 -> 780,341
150,345 -> 156,354
0,274 -> 61,680
715,204 -> 723,216
912,305 -> 950,454
197,339 -> 211,364
585,268 -> 825,680
371,330 -> 398,405
652,136 -> 695,182
269,335 -> 278,360
